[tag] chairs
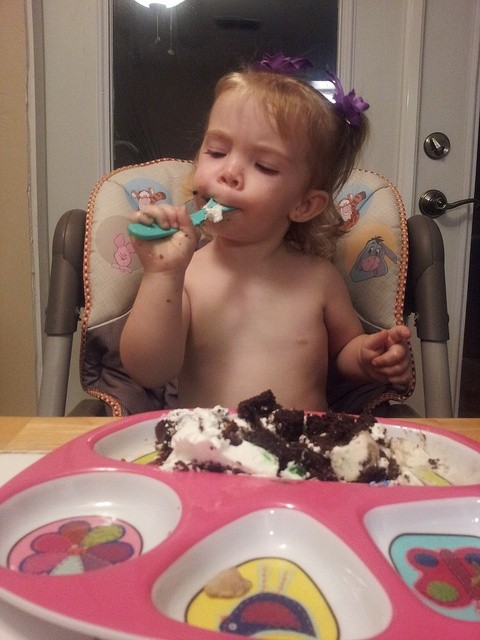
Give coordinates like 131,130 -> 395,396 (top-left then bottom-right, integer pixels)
35,209 -> 454,417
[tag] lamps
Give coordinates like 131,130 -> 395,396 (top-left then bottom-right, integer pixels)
135,1 -> 185,58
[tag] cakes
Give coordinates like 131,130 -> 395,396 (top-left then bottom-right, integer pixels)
155,389 -> 439,487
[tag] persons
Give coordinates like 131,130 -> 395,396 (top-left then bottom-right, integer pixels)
120,58 -> 413,414
